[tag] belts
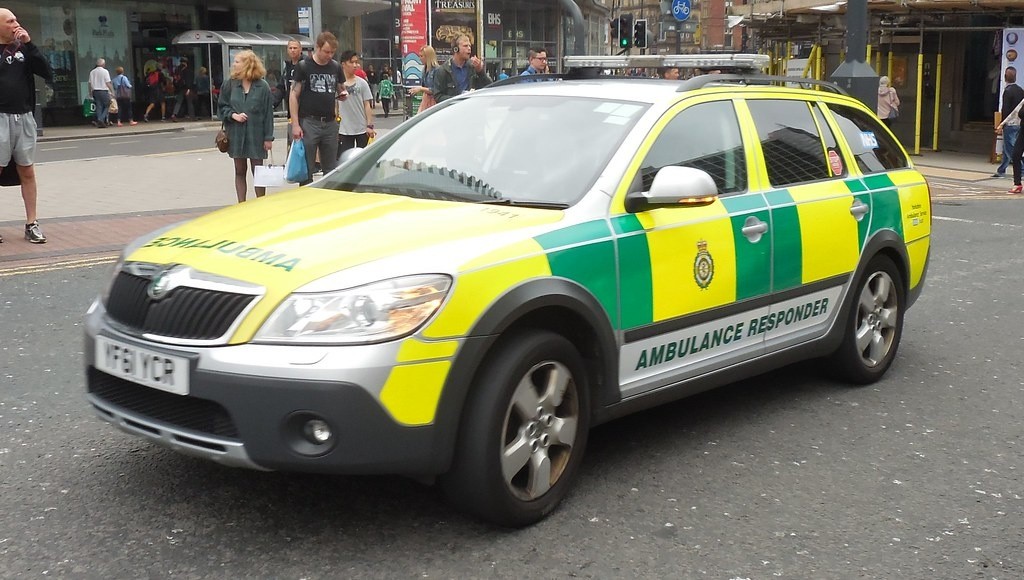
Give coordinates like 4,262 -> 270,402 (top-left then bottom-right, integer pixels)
301,114 -> 336,122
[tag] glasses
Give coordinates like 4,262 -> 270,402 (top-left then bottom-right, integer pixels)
532,57 -> 546,61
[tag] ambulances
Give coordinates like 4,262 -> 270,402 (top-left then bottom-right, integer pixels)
82,52 -> 934,525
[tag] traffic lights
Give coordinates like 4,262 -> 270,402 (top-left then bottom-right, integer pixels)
634,19 -> 647,49
620,14 -> 633,49
610,18 -> 618,38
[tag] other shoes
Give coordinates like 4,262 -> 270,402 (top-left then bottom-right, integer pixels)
172,115 -> 177,121
92,120 -> 98,127
130,121 -> 138,125
142,115 -> 148,122
189,116 -> 196,120
384,110 -> 388,118
97,119 -> 106,127
117,123 -> 123,126
990,173 -> 1004,178
160,117 -> 166,122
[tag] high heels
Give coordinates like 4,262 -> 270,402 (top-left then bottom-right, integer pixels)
1008,183 -> 1023,193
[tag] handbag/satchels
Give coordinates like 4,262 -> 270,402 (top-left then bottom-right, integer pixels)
116,85 -> 132,99
417,91 -> 436,114
887,108 -> 899,123
82,97 -> 97,118
214,121 -> 229,152
254,147 -> 286,187
283,137 -> 308,184
108,98 -> 118,114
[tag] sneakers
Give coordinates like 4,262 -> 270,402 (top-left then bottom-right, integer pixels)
0,236 -> 4,243
24,220 -> 47,244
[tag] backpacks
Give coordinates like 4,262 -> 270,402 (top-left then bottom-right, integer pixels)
144,70 -> 163,89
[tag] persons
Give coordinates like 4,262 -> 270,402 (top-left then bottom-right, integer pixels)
519,46 -> 554,82
171,57 -> 198,122
335,51 -> 378,160
499,68 -> 508,80
990,66 -> 1024,193
217,49 -> 275,203
408,44 -> 439,114
432,35 -> 493,104
376,64 -> 401,118
0,8 -> 52,243
267,60 -> 280,81
878,76 -> 900,128
657,67 -> 680,79
625,67 -> 643,77
111,66 -> 138,126
144,62 -> 169,122
284,39 -> 310,161
289,31 -> 349,187
367,63 -> 378,109
196,67 -> 212,119
354,63 -> 368,82
266,72 -> 278,88
88,57 -> 115,128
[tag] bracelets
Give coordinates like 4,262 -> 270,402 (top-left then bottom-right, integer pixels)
366,125 -> 374,129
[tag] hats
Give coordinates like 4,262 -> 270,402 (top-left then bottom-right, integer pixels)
879,75 -> 891,87
180,57 -> 188,62
